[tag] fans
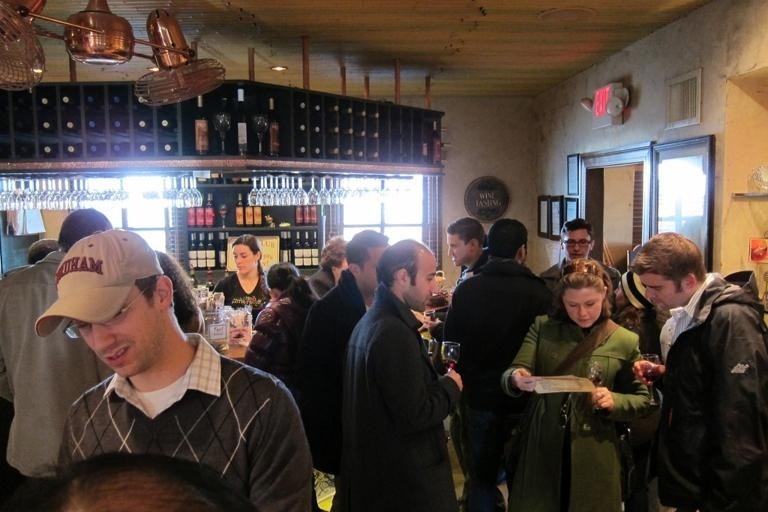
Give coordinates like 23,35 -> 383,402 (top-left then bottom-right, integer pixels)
0,0 -> 231,115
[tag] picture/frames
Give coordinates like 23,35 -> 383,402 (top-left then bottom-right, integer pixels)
535,153 -> 584,244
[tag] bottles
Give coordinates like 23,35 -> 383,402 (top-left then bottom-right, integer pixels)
189,269 -> 232,353
193,94 -> 210,155
187,192 -> 216,228
235,192 -> 317,226
197,178 -> 249,184
232,89 -> 252,156
431,119 -> 441,166
263,96 -> 281,157
296,98 -> 380,159
14,94 -> 175,158
189,231 -> 318,271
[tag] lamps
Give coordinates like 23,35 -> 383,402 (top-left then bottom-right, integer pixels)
575,80 -> 630,131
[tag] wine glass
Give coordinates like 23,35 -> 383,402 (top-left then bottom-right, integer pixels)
638,353 -> 661,406
212,114 -> 232,155
218,203 -> 229,228
0,174 -> 203,212
248,175 -> 401,208
422,309 -> 435,340
251,114 -> 268,156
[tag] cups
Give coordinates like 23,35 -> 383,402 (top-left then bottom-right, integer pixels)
584,361 -> 606,387
441,340 -> 461,372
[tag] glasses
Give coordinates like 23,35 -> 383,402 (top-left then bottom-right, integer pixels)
63,286 -> 149,343
562,238 -> 592,246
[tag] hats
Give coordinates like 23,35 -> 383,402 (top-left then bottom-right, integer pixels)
35,228 -> 165,338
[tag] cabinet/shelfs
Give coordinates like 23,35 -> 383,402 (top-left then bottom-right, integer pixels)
168,178 -> 328,298
